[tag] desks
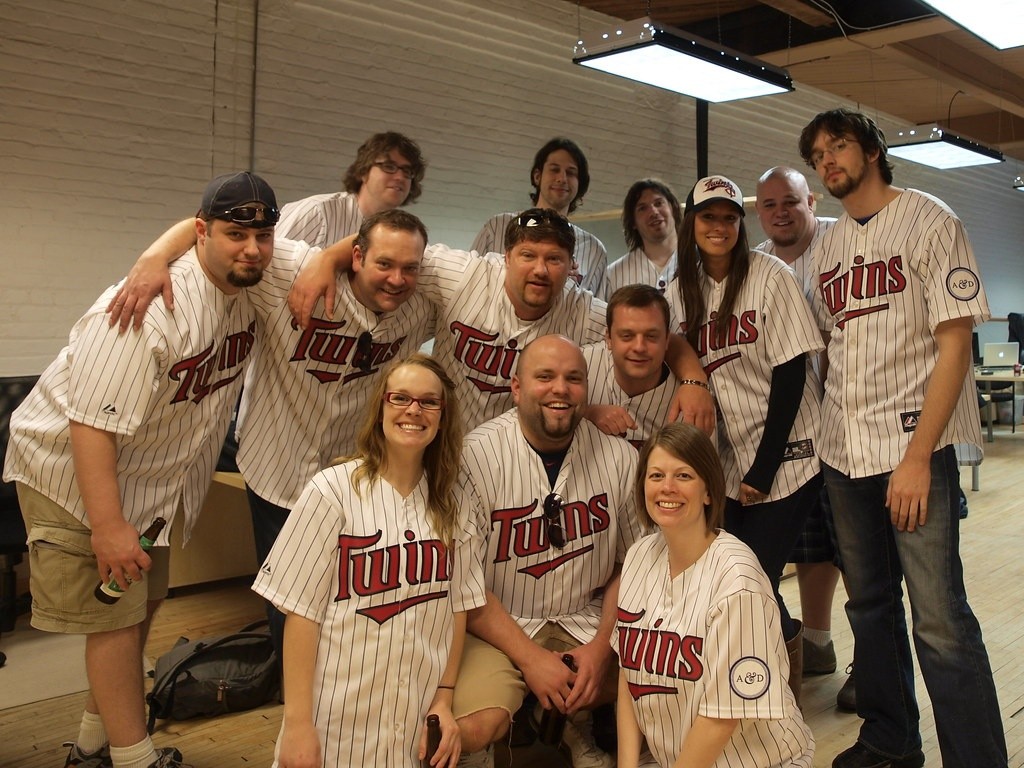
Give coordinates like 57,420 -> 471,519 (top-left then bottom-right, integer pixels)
973,369 -> 1024,442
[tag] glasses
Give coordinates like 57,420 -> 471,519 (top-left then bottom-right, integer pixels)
543,492 -> 570,550
381,391 -> 445,410
371,161 -> 416,179
807,138 -> 865,170
203,207 -> 281,223
508,213 -> 574,236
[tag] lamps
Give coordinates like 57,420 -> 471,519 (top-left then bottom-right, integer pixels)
571,0 -> 795,106
883,37 -> 1006,170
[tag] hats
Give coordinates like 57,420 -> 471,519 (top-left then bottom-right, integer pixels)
195,172 -> 278,227
684,175 -> 745,217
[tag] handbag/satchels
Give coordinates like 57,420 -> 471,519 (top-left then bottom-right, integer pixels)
144,619 -> 280,735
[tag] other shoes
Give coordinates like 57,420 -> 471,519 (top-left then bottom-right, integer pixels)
592,703 -> 619,752
506,704 -> 536,746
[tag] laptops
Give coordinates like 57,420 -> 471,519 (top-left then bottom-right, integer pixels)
976,342 -> 1019,368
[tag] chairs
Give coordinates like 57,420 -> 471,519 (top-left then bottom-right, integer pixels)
976,380 -> 1015,434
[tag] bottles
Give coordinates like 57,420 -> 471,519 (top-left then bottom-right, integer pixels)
538,654 -> 574,746
93,517 -> 166,604
420,715 -> 450,768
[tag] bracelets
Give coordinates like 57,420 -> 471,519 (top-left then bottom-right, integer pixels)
680,380 -> 715,398
437,686 -> 454,689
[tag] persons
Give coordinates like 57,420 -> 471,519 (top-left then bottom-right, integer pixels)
104,209 -> 579,655
798,108 -> 1008,768
2,170 -> 281,768
249,350 -> 490,768
579,283 -> 719,459
753,165 -> 856,711
608,422 -> 816,768
286,208 -> 716,439
594,178 -> 682,304
469,136 -> 608,298
450,332 -> 663,768
662,174 -> 845,721
273,130 -> 426,250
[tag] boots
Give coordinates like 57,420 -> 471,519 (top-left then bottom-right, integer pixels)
785,618 -> 804,718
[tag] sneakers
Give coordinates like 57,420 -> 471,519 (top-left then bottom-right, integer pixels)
455,743 -> 495,768
528,700 -> 617,768
832,742 -> 925,768
62,741 -> 114,768
146,747 -> 195,768
803,636 -> 837,675
837,662 -> 856,712
